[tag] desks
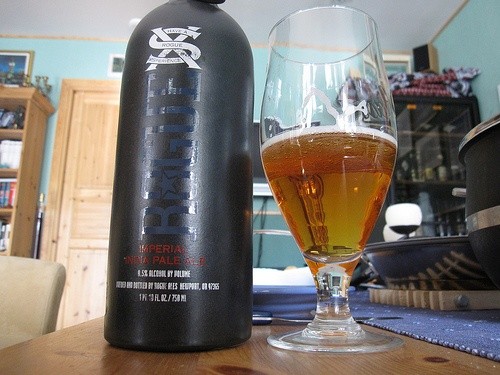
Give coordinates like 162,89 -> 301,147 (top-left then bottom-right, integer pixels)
0,315 -> 500,375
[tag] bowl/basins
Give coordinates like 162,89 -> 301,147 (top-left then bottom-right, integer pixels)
362,237 -> 497,290
458,113 -> 500,289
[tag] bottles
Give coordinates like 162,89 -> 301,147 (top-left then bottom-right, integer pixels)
103,0 -> 253,350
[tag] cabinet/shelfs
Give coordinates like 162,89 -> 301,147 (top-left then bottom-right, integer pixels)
0,85 -> 56,256
366,95 -> 483,244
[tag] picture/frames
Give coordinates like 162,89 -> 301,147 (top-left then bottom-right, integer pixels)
0,49 -> 35,86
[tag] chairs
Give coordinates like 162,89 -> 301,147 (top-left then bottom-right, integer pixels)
1,256 -> 67,351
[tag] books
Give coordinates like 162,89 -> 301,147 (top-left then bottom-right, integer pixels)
0,219 -> 11,252
0,140 -> 23,169
0,182 -> 16,208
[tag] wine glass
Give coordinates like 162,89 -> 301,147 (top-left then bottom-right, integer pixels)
259,5 -> 407,355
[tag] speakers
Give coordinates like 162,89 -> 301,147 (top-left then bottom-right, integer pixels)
411,43 -> 440,73
